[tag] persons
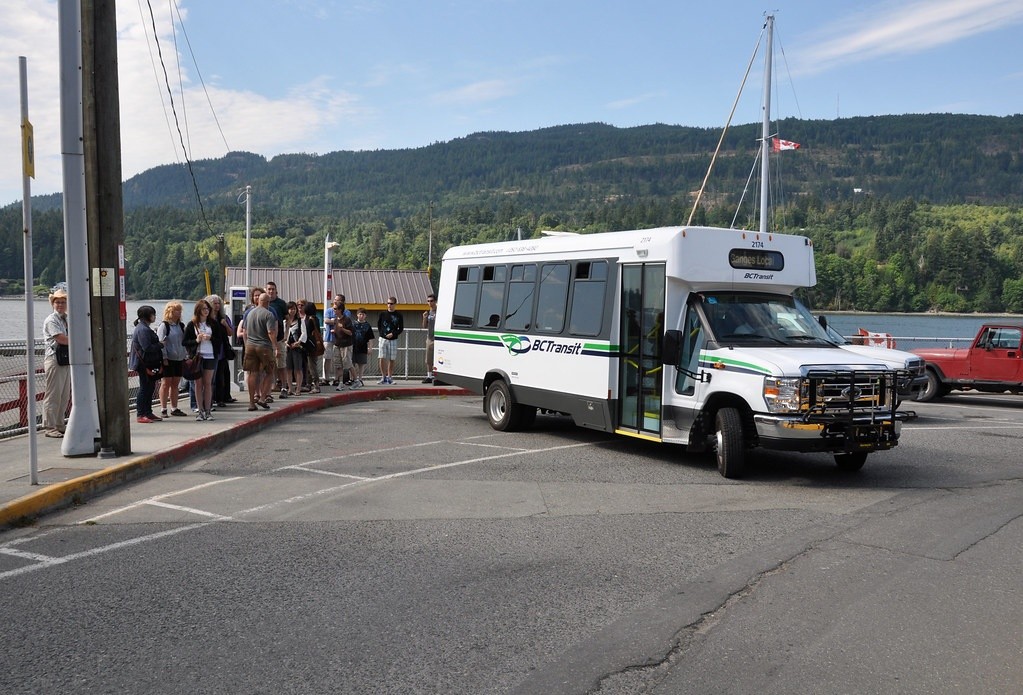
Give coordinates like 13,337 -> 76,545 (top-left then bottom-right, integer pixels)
183,300 -> 223,421
377,297 -> 403,385
156,301 -> 188,417
422,294 -> 437,383
42,289 -> 68,438
330,302 -> 362,391
204,294 -> 238,407
346,308 -> 375,386
237,281 -> 320,403
243,293 -> 277,410
734,303 -> 781,334
128,305 -> 166,423
319,294 -> 351,386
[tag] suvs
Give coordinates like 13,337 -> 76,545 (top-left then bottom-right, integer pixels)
907,321 -> 1023,402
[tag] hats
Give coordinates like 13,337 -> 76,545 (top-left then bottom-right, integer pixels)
49,288 -> 68,306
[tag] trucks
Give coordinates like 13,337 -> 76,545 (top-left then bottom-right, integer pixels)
432,225 -> 929,479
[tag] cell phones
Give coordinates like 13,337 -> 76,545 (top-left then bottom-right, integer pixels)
162,336 -> 168,342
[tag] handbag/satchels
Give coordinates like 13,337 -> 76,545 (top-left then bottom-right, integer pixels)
298,318 -> 317,353
182,353 -> 202,381
146,363 -> 163,377
56,344 -> 70,366
309,317 -> 325,355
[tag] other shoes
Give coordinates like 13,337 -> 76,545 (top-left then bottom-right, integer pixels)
170,408 -> 187,416
422,378 -> 434,383
147,413 -> 162,421
161,409 -> 168,417
57,424 -> 66,433
196,410 -> 204,422
137,416 -> 154,423
193,376 -> 395,412
206,412 -> 214,420
45,429 -> 63,438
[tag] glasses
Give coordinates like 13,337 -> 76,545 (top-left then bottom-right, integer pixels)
334,307 -> 340,310
201,308 -> 210,312
53,300 -> 67,304
387,303 -> 395,305
427,300 -> 433,302
298,304 -> 305,307
